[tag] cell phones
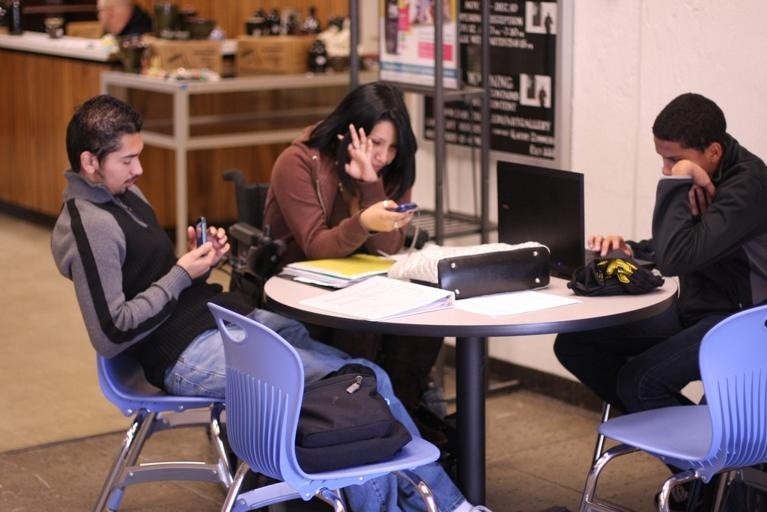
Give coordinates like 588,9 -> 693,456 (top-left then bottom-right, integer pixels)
195,216 -> 208,256
394,204 -> 417,214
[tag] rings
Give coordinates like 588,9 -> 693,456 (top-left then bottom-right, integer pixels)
384,200 -> 389,208
393,221 -> 399,228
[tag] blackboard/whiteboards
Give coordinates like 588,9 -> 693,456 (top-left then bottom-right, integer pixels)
414,0 -> 574,171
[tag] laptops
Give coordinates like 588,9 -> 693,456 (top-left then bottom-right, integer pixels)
497,160 -> 657,281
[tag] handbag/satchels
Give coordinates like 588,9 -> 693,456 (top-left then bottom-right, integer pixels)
387,238 -> 551,299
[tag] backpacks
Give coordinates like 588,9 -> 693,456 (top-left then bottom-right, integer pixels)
293,360 -> 413,474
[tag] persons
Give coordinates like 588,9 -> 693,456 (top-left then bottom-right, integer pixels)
50,93 -> 491,512
97,1 -> 154,40
259,81 -> 448,447
553,92 -> 767,512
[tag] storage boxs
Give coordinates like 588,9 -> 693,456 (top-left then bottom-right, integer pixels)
236,37 -> 314,77
150,38 -> 221,73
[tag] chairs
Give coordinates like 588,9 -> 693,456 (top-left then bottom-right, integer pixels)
205,300 -> 442,512
92,348 -> 236,512
577,304 -> 767,512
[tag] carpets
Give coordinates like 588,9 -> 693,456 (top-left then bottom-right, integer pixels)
0,215 -> 171,455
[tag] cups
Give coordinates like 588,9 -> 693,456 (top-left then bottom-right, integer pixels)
5,4 -> 23,35
45,18 -> 64,39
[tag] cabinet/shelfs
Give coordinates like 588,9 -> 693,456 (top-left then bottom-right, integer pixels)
350,1 -> 491,245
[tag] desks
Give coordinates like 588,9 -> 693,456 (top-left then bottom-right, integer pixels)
98,69 -> 377,260
263,242 -> 678,505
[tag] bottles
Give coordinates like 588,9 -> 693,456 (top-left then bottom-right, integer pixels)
307,40 -> 328,74
303,5 -> 319,35
